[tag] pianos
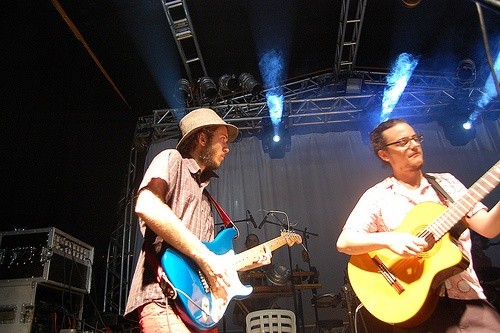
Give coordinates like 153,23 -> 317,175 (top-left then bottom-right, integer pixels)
233,269 -> 322,314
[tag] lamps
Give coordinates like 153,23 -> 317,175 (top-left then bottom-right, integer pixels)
262,117 -> 291,160
199,77 -> 218,99
345,77 -> 363,95
173,79 -> 193,104
442,113 -> 476,146
218,74 -> 239,90
456,58 -> 476,84
239,73 -> 262,96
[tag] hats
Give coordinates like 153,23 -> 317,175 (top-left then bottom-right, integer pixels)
175,108 -> 238,150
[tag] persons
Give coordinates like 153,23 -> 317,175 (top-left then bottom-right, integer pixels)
336,119 -> 500,332
470,230 -> 493,273
124,108 -> 273,333
232,234 -> 272,333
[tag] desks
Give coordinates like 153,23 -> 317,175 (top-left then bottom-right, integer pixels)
235,284 -> 322,328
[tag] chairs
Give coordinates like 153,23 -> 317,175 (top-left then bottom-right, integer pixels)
246,309 -> 296,333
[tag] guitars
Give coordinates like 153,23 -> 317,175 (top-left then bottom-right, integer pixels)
161,227 -> 303,330
348,161 -> 500,328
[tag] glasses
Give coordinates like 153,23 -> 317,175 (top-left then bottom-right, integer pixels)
386,135 -> 424,146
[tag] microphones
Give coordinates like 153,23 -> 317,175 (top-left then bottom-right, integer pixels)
248,210 -> 257,228
258,213 -> 269,229
